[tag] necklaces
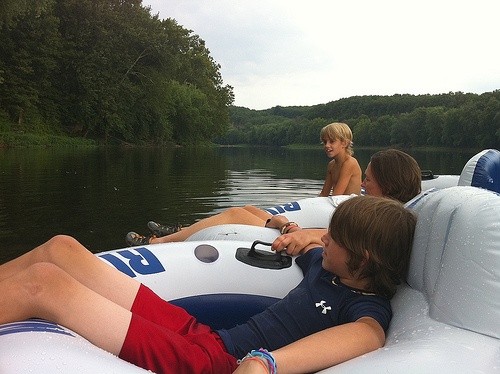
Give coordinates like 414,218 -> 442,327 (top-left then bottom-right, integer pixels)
332,276 -> 377,295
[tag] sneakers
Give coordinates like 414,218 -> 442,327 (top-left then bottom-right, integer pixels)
126,221 -> 183,246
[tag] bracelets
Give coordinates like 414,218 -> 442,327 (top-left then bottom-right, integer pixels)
280,221 -> 299,234
236,348 -> 277,374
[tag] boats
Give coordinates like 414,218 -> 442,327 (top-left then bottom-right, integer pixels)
184,148 -> 500,243
0,186 -> 500,373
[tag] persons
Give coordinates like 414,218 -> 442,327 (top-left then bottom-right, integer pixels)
320,123 -> 362,197
126,149 -> 421,256
0,196 -> 416,374
